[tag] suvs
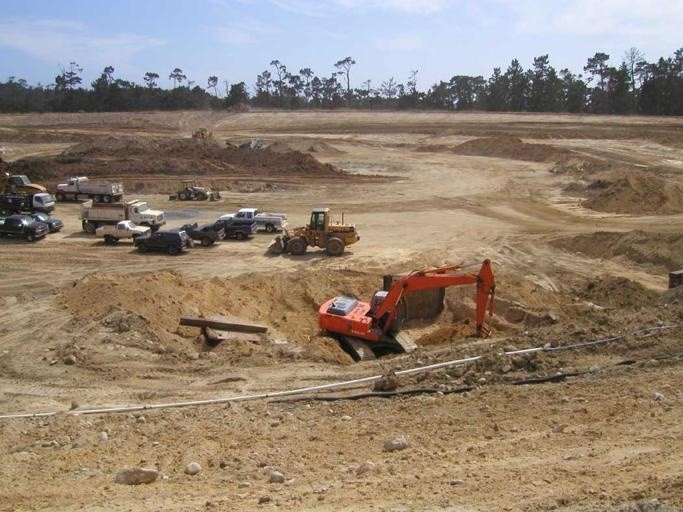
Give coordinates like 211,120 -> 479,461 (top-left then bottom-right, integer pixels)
134,230 -> 194,255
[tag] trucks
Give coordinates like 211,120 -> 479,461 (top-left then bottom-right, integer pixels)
0,173 -> 166,234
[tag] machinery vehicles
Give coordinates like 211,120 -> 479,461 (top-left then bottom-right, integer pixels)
177,180 -> 208,200
267,208 -> 360,257
318,259 -> 494,360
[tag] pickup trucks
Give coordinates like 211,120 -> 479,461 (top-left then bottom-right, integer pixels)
181,208 -> 288,246
96,220 -> 151,244
0,214 -> 50,241
